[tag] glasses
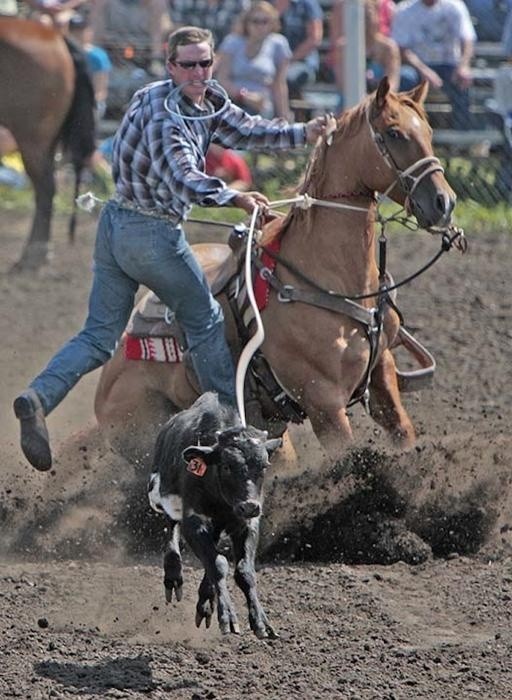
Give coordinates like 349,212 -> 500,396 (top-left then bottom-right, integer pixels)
174,58 -> 214,70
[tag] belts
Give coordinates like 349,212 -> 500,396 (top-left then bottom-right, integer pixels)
112,194 -> 171,223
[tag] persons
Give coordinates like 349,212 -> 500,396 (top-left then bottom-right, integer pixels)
12,25 -> 339,472
0,1 -> 512,194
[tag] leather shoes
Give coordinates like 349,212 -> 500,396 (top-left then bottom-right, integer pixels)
12,389 -> 52,472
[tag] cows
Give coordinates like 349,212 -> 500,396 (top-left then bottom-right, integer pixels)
146,386 -> 286,644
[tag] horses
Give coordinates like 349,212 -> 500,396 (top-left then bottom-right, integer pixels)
46,72 -> 459,499
0,10 -> 98,279
